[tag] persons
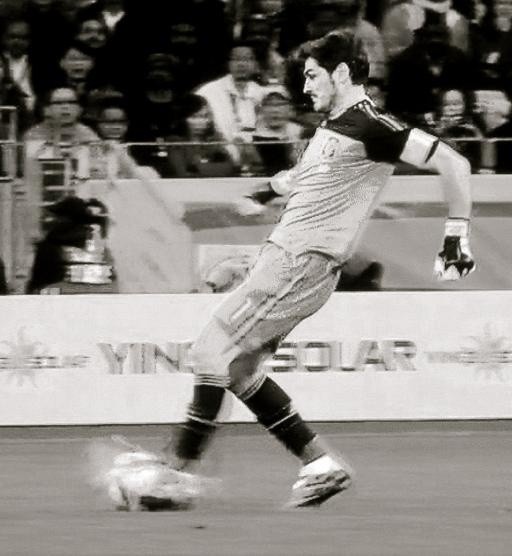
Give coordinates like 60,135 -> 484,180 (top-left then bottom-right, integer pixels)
107,27 -> 478,514
0,0 -> 512,179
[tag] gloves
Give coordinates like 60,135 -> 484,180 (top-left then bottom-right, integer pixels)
243,180 -> 283,205
432,218 -> 476,281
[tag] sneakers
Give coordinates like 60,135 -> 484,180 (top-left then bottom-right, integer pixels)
139,471 -> 192,511
283,468 -> 352,508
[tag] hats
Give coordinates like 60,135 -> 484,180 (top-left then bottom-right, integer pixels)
258,84 -> 292,102
